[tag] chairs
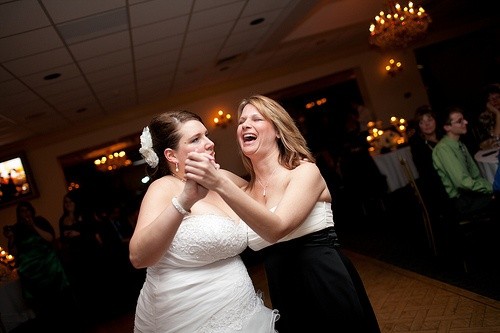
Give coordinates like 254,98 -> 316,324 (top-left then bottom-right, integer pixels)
397,155 -> 497,272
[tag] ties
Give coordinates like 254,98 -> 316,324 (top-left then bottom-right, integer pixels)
114,222 -> 121,231
459,144 -> 472,175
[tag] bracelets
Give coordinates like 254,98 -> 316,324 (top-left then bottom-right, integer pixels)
171,195 -> 192,217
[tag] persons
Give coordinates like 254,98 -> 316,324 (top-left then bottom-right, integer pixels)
10,192 -> 126,333
411,81 -> 500,237
184,94 -> 381,333
130,111 -> 280,333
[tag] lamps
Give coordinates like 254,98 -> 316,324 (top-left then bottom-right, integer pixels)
385,59 -> 403,77
368,0 -> 433,51
213,110 -> 233,129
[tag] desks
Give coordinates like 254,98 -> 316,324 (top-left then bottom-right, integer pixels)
474,147 -> 500,184
0,268 -> 36,333
370,146 -> 419,193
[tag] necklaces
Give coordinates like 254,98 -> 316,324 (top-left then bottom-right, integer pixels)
255,161 -> 285,197
172,173 -> 186,183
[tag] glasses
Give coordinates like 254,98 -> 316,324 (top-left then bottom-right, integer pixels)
451,116 -> 466,124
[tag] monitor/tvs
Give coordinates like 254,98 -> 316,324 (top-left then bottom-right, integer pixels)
0,151 -> 40,211
